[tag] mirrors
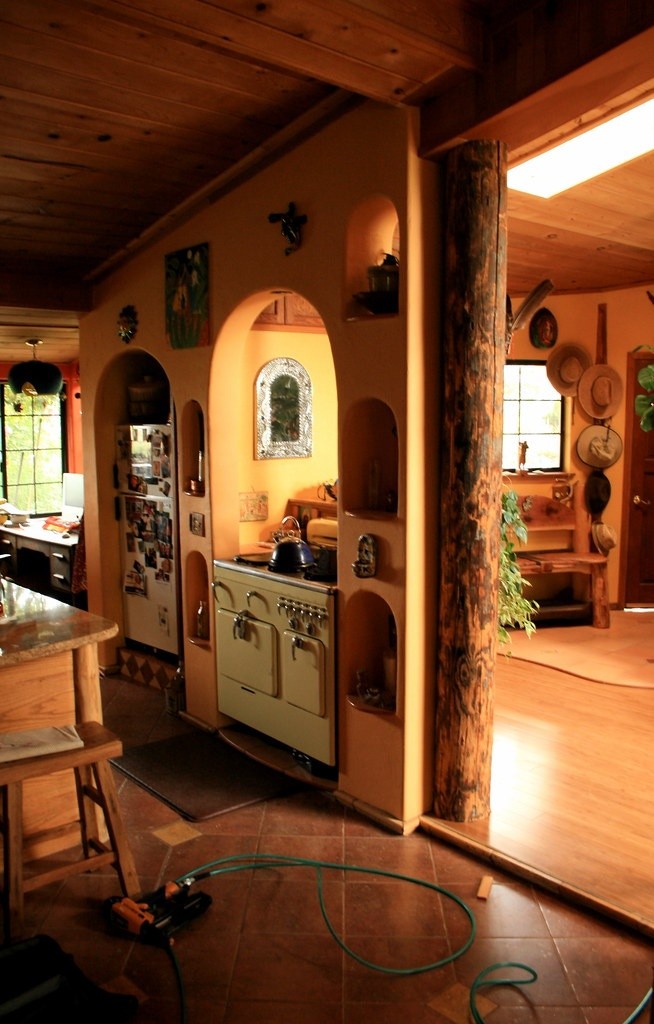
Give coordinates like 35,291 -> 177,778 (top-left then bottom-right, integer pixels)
252,357 -> 313,460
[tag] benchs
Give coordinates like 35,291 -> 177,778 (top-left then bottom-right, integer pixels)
516,552 -> 611,630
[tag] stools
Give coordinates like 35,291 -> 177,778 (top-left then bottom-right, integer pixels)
0,720 -> 141,920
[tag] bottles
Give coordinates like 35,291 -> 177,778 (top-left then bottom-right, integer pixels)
197,601 -> 208,638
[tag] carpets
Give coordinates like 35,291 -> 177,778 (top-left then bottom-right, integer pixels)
496,609 -> 654,689
108,731 -> 309,823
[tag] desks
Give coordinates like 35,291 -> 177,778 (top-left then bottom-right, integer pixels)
0,518 -> 88,609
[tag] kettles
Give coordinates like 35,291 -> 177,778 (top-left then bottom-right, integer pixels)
268,515 -> 315,571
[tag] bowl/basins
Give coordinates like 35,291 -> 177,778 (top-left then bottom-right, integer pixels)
10,513 -> 27,529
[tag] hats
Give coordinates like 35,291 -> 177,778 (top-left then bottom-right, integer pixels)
578,364 -> 624,421
546,344 -> 594,397
592,521 -> 615,556
577,423 -> 623,469
584,470 -> 611,514
529,307 -> 558,348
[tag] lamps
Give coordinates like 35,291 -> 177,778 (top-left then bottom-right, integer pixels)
7,339 -> 64,396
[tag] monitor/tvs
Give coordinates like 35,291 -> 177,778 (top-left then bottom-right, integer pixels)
62,473 -> 84,522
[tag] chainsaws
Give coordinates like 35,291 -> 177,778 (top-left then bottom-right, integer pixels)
103,880 -> 214,949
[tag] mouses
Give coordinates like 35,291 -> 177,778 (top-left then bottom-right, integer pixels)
62,533 -> 70,538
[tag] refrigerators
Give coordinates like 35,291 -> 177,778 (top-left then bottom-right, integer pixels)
113,424 -> 184,670
